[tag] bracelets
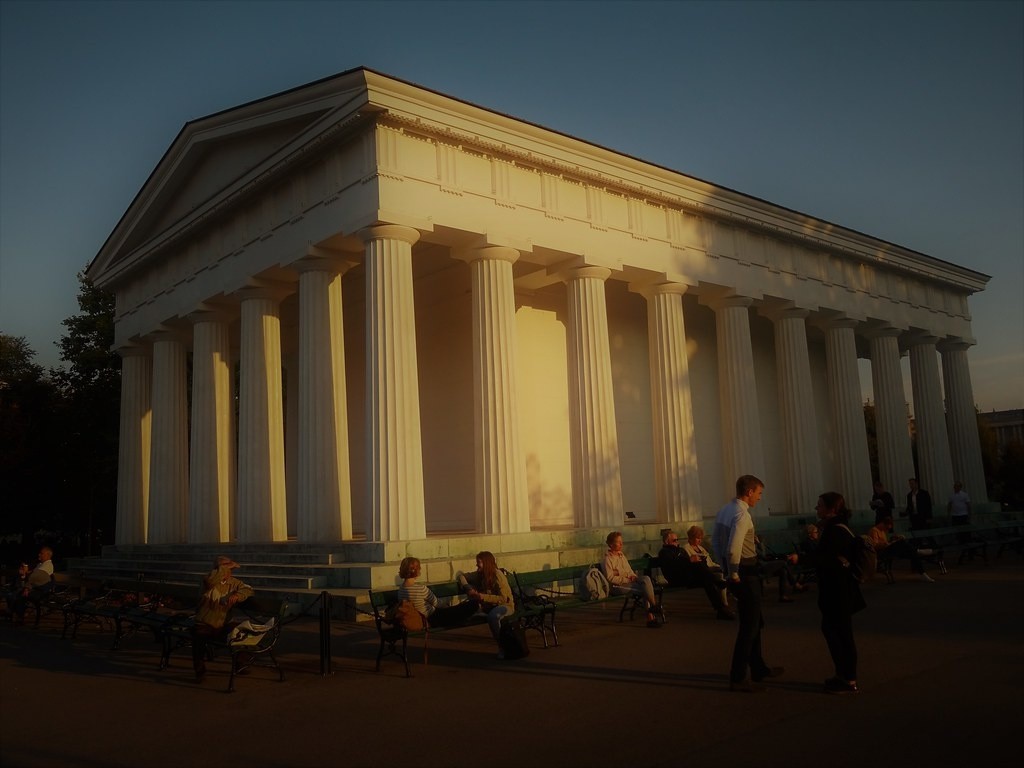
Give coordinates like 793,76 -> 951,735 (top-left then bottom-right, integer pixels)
25,588 -> 28,591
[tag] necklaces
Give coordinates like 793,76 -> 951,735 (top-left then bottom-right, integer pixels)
220,580 -> 226,584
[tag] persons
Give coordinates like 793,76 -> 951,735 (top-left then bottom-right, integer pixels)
4,546 -> 55,623
803,517 -> 936,585
191,554 -> 251,682
658,526 -> 737,622
754,533 -> 809,604
400,550 -> 515,661
868,477 -> 979,570
787,492 -> 860,693
712,474 -> 786,694
602,532 -> 664,631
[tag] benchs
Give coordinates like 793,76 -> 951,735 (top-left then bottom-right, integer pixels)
368,568 -> 551,679
8,571 -> 80,641
117,574 -> 198,668
68,571 -> 141,650
160,590 -> 291,694
514,519 -> 1024,648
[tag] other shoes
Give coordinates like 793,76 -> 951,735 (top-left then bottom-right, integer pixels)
754,681 -> 772,691
734,681 -> 753,695
195,668 -> 207,684
826,677 -> 860,694
916,548 -> 933,556
647,620 -> 662,628
496,648 -> 503,660
648,603 -> 660,617
919,573 -> 935,584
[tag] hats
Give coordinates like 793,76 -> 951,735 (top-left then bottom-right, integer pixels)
215,556 -> 240,568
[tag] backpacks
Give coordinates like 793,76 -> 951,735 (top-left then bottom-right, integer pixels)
578,561 -> 610,601
834,523 -> 879,585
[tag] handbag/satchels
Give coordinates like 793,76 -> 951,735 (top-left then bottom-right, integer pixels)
394,598 -> 424,632
500,622 -> 530,660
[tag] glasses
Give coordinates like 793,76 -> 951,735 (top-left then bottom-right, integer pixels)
671,538 -> 679,542
